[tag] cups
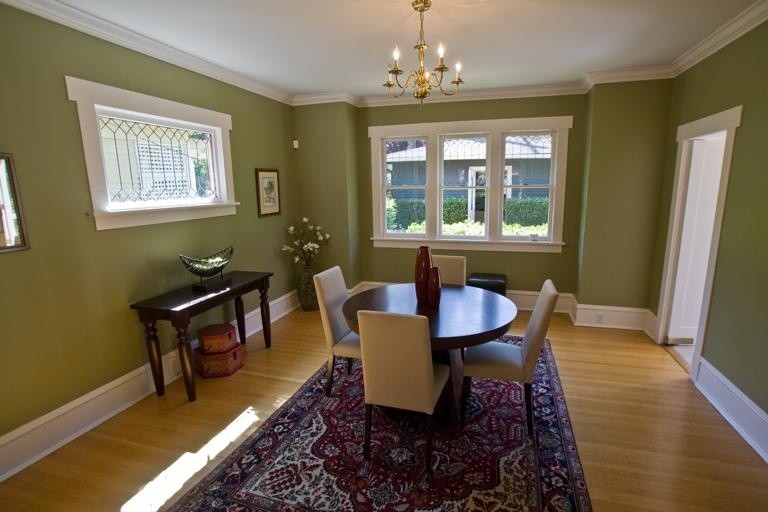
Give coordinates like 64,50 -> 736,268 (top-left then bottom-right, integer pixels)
530,233 -> 538,241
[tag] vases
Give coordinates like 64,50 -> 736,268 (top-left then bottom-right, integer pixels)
296,266 -> 319,310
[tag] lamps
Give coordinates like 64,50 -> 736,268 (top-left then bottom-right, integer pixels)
382,0 -> 462,106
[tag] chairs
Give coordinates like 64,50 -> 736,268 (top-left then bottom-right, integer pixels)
432,255 -> 466,285
356,310 -> 450,470
313,265 -> 362,397
460,278 -> 558,435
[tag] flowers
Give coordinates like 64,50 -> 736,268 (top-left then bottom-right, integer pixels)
281,218 -> 330,265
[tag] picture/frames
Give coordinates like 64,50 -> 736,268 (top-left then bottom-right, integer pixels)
0,152 -> 30,253
255,166 -> 281,216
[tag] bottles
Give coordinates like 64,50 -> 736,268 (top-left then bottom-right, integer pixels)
429,268 -> 441,311
415,246 -> 433,304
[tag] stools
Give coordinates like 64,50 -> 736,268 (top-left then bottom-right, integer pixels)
467,273 -> 506,295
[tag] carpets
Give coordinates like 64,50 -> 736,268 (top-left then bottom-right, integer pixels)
166,337 -> 593,512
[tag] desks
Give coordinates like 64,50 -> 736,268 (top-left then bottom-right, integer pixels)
342,284 -> 517,427
129,270 -> 274,402
